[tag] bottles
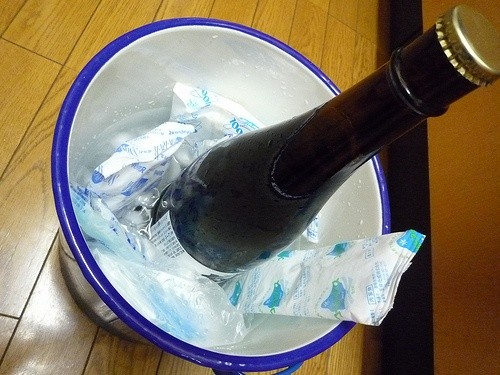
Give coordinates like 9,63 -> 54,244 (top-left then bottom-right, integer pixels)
147,3 -> 499,288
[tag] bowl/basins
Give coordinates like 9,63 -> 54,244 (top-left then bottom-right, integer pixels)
49,15 -> 392,375
